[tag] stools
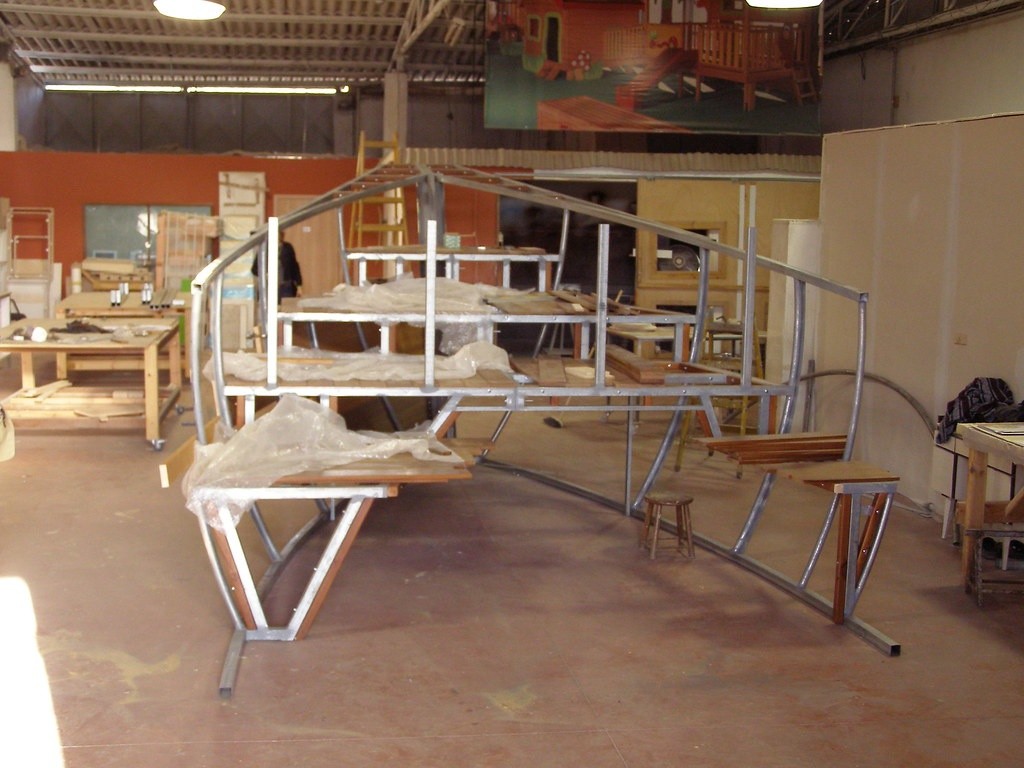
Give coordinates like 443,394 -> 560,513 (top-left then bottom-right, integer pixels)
965,523 -> 1024,607
640,492 -> 694,559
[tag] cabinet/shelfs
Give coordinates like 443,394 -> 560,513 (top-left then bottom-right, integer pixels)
930,414 -> 1024,573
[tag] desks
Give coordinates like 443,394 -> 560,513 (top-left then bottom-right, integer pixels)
0,317 -> 183,451
955,422 -> 1024,595
55,292 -> 206,379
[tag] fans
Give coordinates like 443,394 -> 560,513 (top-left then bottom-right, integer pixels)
657,245 -> 704,274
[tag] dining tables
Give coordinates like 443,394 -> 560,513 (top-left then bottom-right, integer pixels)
608,326 -> 767,360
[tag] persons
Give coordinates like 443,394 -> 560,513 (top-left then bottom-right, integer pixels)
250,229 -> 302,307
